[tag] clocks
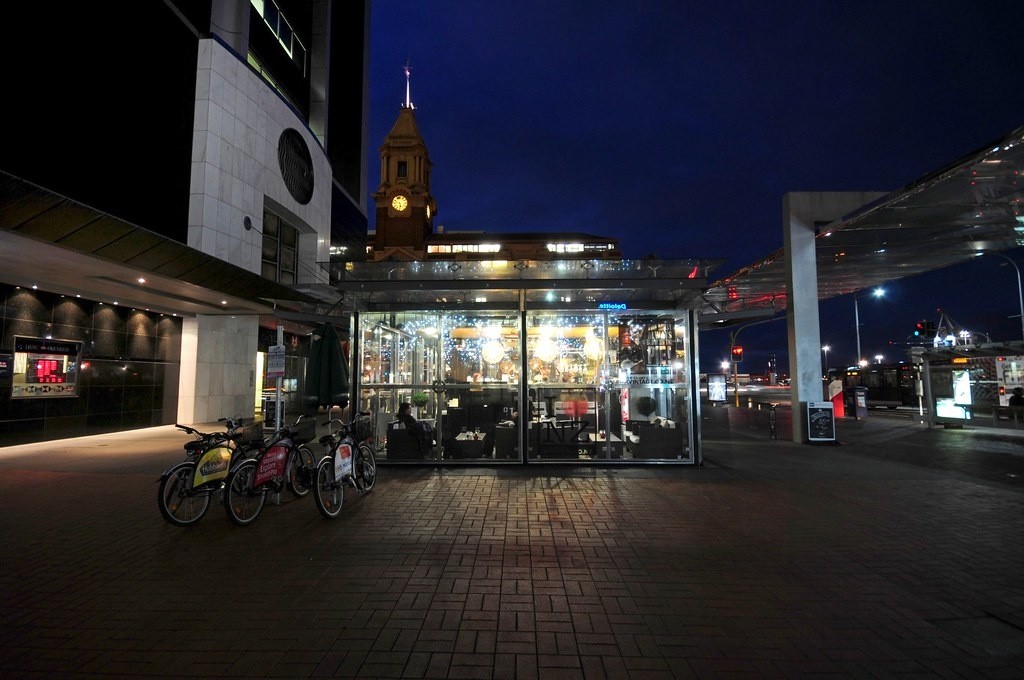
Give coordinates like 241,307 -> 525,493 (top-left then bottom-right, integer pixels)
391,195 -> 408,211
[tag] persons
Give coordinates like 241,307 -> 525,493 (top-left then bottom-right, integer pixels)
396,403 -> 433,452
1009,388 -> 1024,419
871,381 -> 877,387
513,400 -> 536,424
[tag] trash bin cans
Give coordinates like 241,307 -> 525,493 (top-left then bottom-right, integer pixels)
846,385 -> 868,417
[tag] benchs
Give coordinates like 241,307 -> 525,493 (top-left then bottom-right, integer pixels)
954,403 -> 1024,430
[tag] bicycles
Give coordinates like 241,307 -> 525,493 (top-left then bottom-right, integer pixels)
224,411 -> 317,525
312,411 -> 377,519
154,415 -> 267,526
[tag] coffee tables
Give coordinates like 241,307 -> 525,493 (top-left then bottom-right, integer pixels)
587,433 -> 623,459
453,433 -> 488,458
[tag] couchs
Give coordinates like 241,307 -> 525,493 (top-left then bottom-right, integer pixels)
495,419 -> 538,459
386,419 -> 437,459
539,417 -> 579,458
624,419 -> 683,459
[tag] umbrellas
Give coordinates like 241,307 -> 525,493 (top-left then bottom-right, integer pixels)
304,322 -> 350,456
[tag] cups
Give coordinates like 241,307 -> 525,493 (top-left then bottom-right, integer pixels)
461,427 -> 467,433
600,429 -> 605,438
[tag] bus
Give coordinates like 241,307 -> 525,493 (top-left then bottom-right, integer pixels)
827,362 -> 923,409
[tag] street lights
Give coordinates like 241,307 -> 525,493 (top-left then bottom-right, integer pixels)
976,251 -> 1024,342
822,345 -> 830,370
854,288 -> 885,366
731,315 -> 786,407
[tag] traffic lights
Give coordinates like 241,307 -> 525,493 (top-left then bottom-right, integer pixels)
917,322 -> 925,335
731,346 -> 743,362
928,322 -> 935,336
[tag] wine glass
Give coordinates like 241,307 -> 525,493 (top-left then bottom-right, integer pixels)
475,427 -> 480,437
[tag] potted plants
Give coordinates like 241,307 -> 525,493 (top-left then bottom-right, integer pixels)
411,392 -> 429,408
